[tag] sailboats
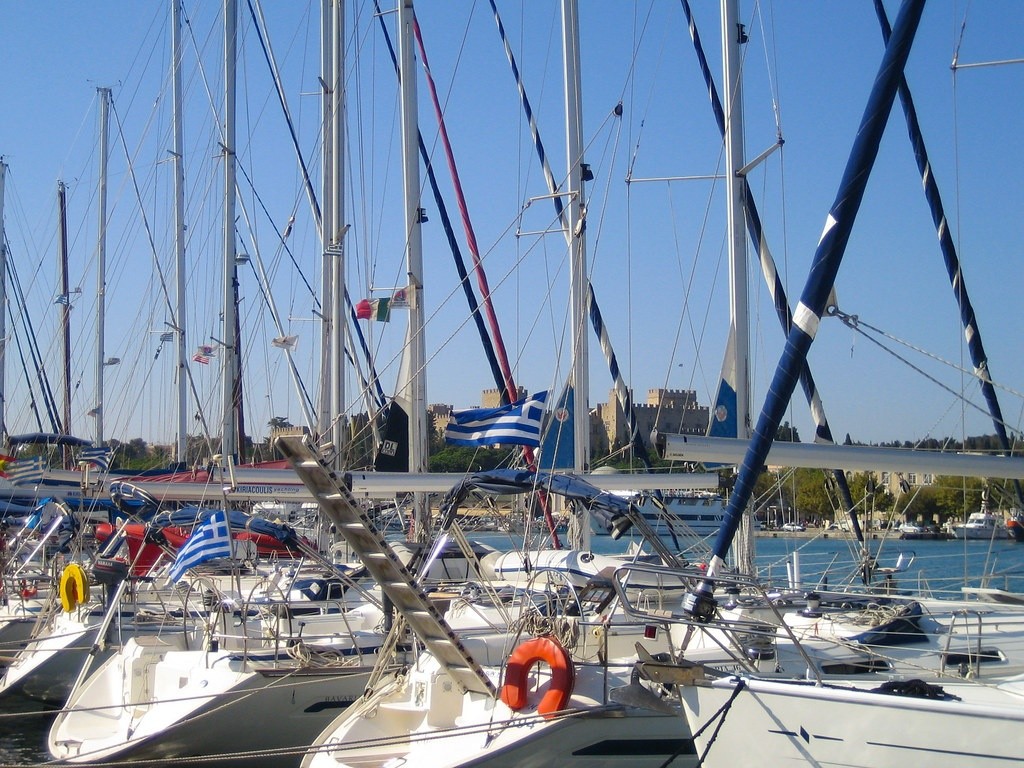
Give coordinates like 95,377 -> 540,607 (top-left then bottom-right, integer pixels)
0,0 -> 1022,768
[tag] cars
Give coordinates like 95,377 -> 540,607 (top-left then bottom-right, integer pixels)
781,522 -> 806,532
899,523 -> 923,533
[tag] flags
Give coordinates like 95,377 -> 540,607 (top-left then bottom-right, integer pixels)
160,332 -> 173,342
6,455 -> 47,486
387,284 -> 416,309
200,343 -> 220,357
445,390 -> 548,447
271,335 -> 298,351
192,352 -> 209,364
169,510 -> 233,583
0,454 -> 16,478
356,297 -> 390,322
78,446 -> 117,470
